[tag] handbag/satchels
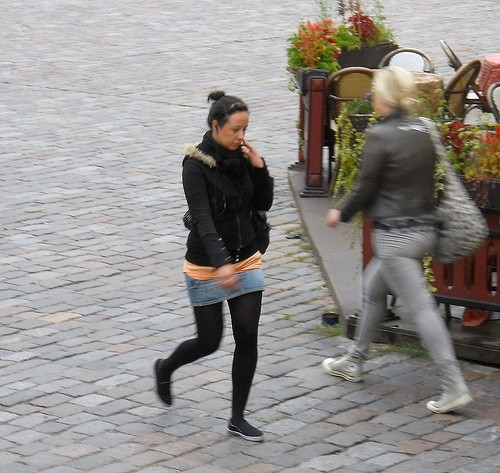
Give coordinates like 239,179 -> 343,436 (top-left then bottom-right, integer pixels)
251,209 -> 272,254
418,116 -> 489,264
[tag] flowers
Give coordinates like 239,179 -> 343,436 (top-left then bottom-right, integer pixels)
285,0 -> 395,92
440,121 -> 500,175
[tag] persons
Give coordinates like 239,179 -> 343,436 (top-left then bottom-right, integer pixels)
154,91 -> 274,442
323,64 -> 473,412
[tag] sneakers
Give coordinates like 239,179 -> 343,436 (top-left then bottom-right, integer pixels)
425,382 -> 473,413
322,358 -> 364,382
153,358 -> 174,406
227,419 -> 265,441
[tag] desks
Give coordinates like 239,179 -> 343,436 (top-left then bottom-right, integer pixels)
477,53 -> 500,95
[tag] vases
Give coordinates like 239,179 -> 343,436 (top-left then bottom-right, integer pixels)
460,175 -> 500,211
337,42 -> 398,69
295,70 -> 329,90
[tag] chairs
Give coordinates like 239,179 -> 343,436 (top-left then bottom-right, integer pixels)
486,82 -> 500,123
326,67 -> 373,184
378,47 -> 435,73
440,40 -> 492,113
444,59 -> 481,119
